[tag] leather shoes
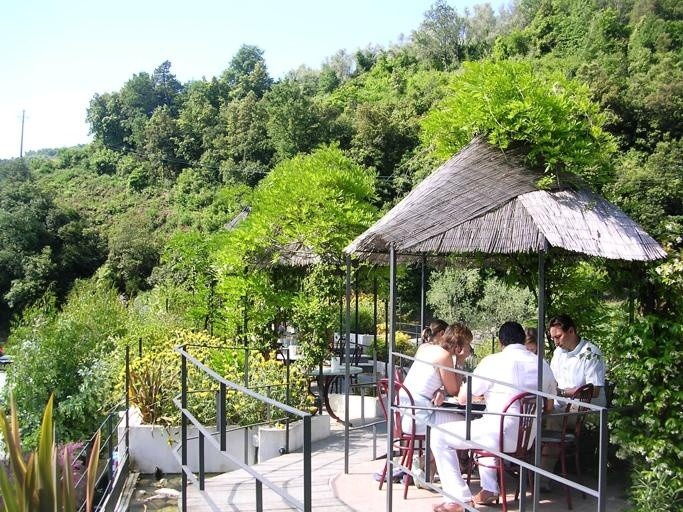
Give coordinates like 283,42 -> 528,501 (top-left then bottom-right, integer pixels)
540,482 -> 551,493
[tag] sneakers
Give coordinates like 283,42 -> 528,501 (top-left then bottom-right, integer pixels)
412,456 -> 426,489
432,488 -> 499,512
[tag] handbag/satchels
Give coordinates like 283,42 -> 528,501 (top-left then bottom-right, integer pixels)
374,466 -> 408,482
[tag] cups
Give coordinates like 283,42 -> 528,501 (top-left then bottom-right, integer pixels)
331,356 -> 340,373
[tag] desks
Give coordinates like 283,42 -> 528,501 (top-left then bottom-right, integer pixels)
302,366 -> 362,427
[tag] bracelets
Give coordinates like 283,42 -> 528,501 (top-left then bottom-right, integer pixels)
560,389 -> 566,397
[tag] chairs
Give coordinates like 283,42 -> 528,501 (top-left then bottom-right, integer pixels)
336,346 -> 364,393
377,366 -> 595,512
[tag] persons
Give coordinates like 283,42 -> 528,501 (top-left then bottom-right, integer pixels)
522,325 -> 539,356
427,321 -> 557,512
423,319 -> 449,346
526,313 -> 607,494
390,323 -> 470,489
